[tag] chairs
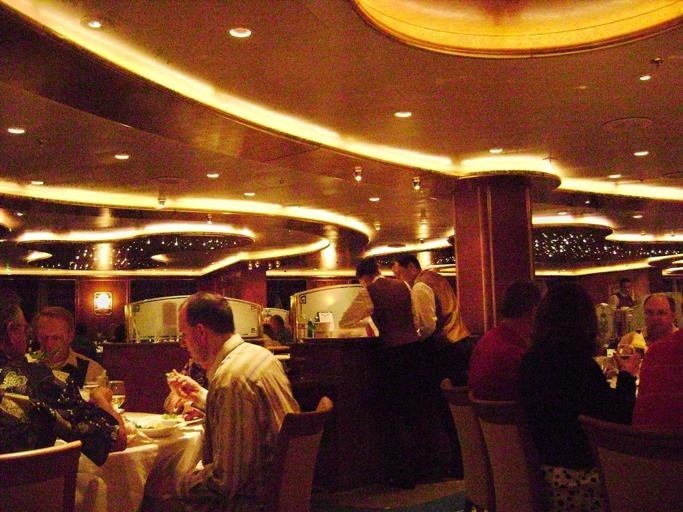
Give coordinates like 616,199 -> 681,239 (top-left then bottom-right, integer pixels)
1,396 -> 333,512
441,376 -> 682,511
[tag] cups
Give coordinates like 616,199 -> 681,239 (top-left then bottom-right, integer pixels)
81,381 -> 126,416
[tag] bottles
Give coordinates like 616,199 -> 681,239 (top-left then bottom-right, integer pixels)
307,321 -> 314,338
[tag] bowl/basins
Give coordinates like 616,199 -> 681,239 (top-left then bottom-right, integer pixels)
136,421 -> 186,437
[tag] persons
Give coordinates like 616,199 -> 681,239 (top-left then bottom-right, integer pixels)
339,256 -> 419,490
390,254 -> 473,478
